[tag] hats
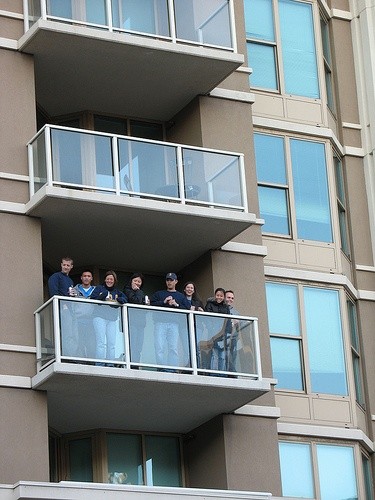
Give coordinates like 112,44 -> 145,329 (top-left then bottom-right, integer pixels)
166,273 -> 178,280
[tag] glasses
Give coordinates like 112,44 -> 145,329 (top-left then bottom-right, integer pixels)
167,278 -> 175,281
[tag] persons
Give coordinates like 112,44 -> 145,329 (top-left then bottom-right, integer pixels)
204,287 -> 232,378
73,270 -> 98,359
90,271 -> 128,367
151,273 -> 191,374
180,282 -> 206,375
48,257 -> 79,364
123,273 -> 150,370
224,290 -> 241,379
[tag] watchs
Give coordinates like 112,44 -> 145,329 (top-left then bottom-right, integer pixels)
176,304 -> 179,307
